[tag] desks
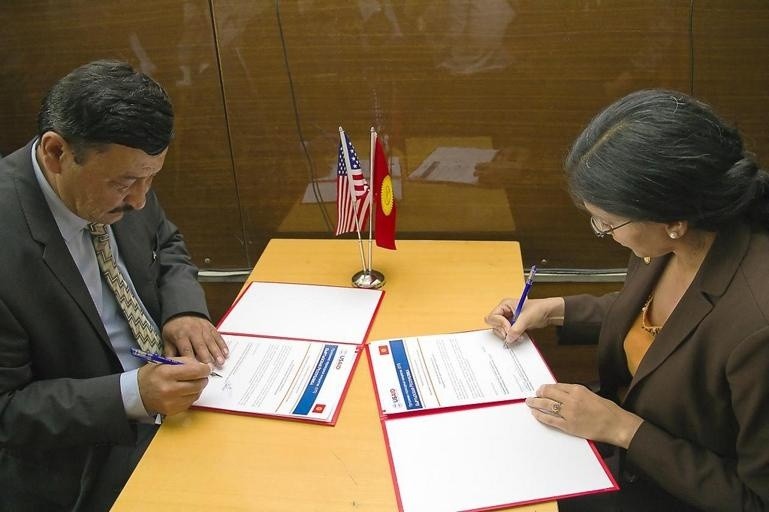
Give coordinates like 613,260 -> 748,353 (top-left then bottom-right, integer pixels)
107,238 -> 560,512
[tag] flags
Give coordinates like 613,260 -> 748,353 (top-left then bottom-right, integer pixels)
336,132 -> 370,236
373,132 -> 397,250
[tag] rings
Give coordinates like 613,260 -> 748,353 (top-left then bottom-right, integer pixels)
551,402 -> 561,413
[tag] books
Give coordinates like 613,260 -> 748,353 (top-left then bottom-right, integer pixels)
366,328 -> 621,512
189,281 -> 386,427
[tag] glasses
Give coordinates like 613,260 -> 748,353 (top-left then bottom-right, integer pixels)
590,215 -> 632,238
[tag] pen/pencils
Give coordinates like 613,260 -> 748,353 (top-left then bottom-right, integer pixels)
130,347 -> 223,378
503,265 -> 537,349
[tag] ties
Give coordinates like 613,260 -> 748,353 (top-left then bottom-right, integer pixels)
86,222 -> 165,362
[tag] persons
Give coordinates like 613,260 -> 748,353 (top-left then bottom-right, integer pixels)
485,89 -> 769,512
0,62 -> 230,512
